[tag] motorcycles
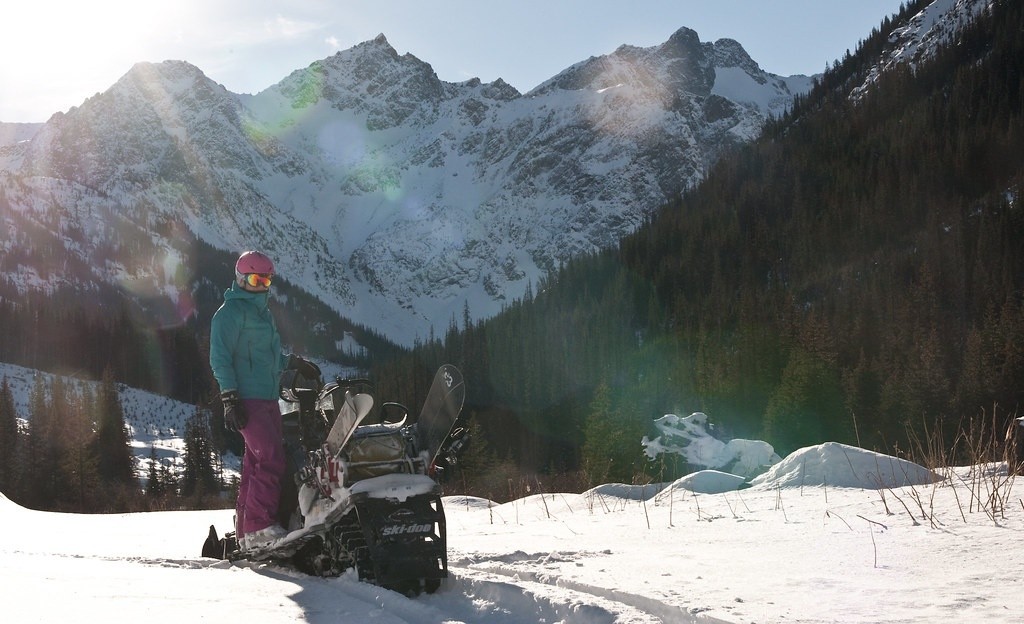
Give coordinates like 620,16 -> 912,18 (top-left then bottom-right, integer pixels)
201,356 -> 472,595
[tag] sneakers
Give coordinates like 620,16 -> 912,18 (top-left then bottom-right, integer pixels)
238,538 -> 246,552
244,524 -> 291,550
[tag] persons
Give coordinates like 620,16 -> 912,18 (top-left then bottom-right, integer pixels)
210,250 -> 322,550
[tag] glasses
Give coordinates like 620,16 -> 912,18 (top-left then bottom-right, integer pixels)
246,274 -> 272,287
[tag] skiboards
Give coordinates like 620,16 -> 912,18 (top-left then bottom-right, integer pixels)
293,389 -> 375,517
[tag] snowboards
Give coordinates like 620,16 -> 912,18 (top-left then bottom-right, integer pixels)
417,364 -> 471,476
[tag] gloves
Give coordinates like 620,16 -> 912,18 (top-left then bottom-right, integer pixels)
300,359 -> 321,379
221,391 -> 247,432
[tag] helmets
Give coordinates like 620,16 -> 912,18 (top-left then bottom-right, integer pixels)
235,251 -> 274,280
